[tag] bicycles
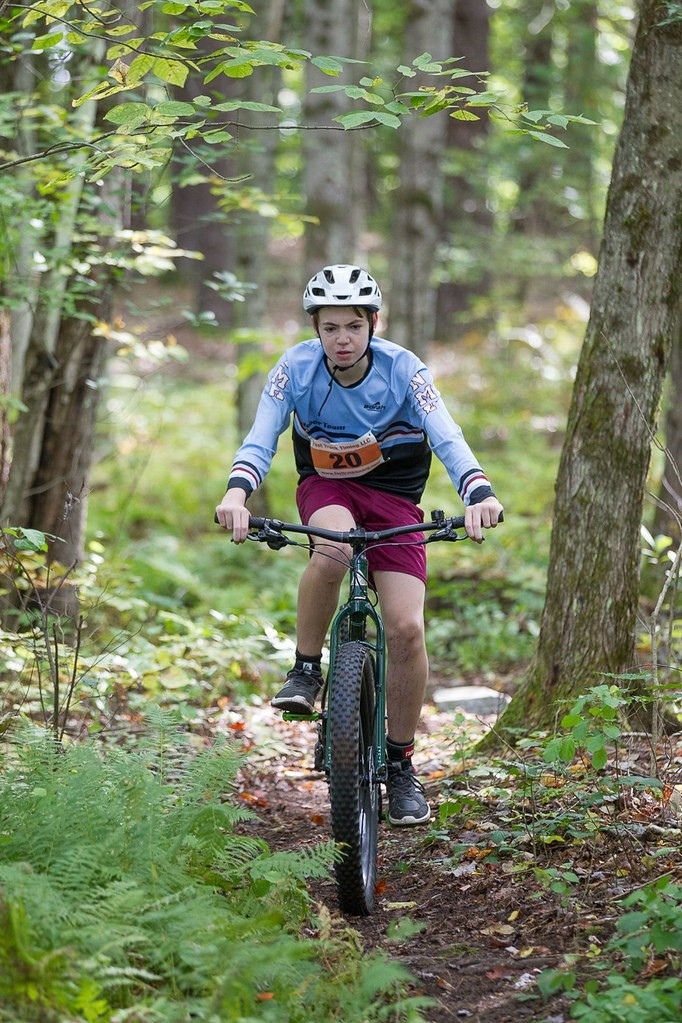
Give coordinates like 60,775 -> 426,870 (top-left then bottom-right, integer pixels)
213,508 -> 503,922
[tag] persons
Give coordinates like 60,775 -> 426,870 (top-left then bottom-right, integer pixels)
215,264 -> 504,825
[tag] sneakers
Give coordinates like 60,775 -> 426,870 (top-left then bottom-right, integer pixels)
385,758 -> 431,825
271,661 -> 324,715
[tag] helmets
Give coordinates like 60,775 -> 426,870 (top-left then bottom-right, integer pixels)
303,264 -> 383,315
